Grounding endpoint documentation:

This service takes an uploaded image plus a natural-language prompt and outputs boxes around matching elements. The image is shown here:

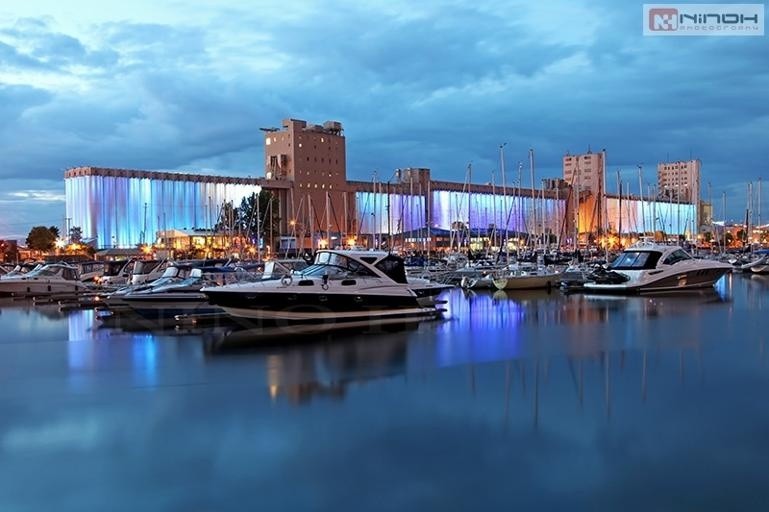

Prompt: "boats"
[0,141,769,338]
